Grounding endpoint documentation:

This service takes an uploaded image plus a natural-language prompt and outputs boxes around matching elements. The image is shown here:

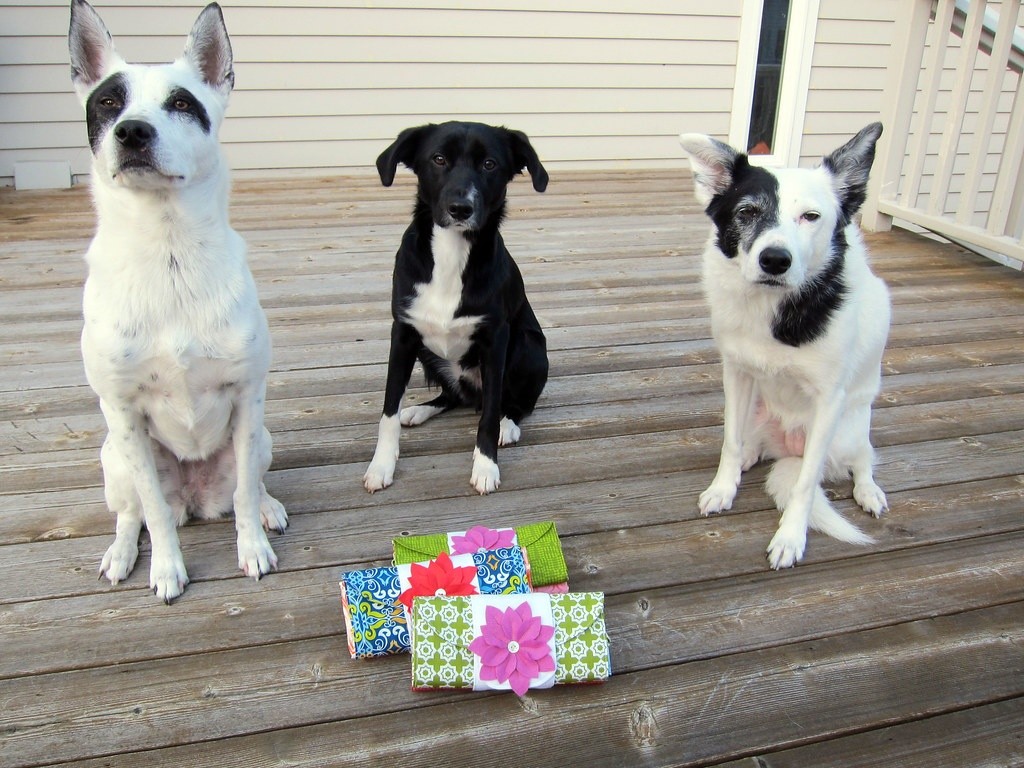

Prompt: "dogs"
[65,1,291,603]
[673,122,891,572]
[358,121,551,495]
